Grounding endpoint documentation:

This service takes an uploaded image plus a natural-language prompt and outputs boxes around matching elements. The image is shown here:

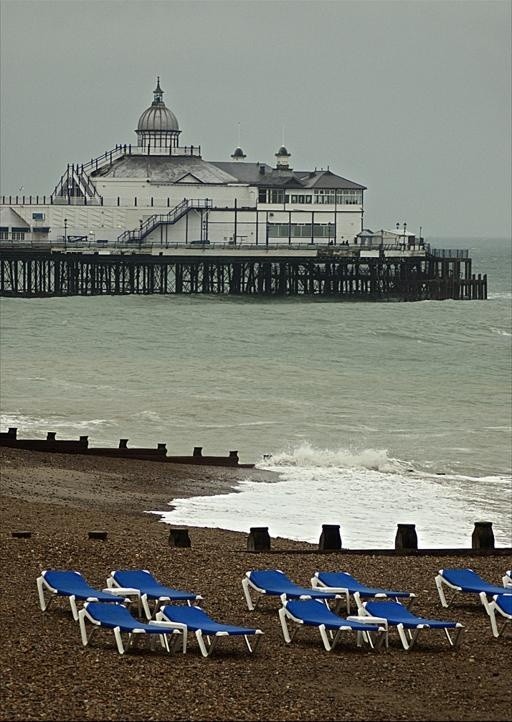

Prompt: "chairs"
[241,569,466,652]
[36,570,265,658]
[435,567,512,640]
